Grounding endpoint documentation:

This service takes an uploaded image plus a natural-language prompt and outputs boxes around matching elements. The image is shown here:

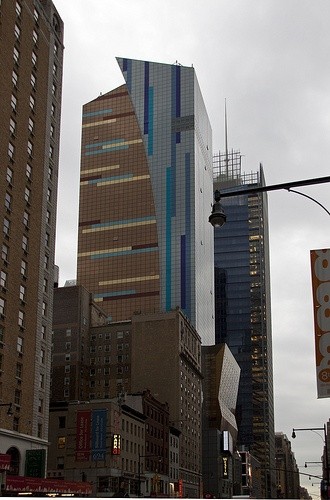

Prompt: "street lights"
[291,424,329,472]
[139,454,163,497]
